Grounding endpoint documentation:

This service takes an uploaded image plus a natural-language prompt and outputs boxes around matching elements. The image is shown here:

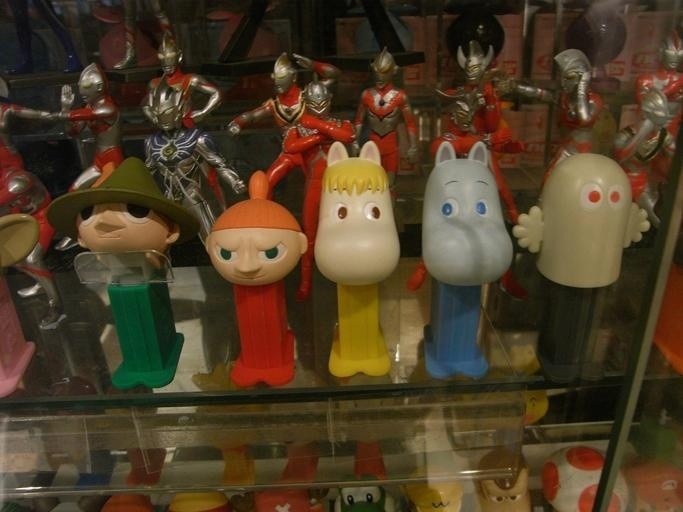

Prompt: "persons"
[1,1,683,301]
